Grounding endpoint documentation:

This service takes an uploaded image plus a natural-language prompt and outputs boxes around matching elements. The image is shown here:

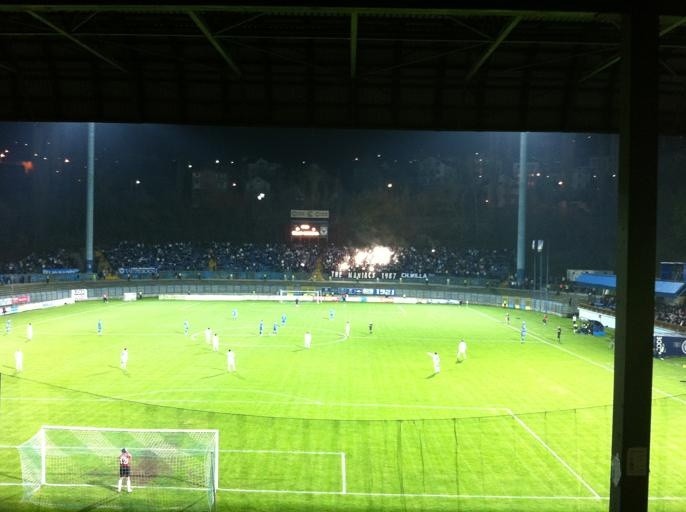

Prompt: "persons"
[117,448,133,493]
[0,240,686,376]
